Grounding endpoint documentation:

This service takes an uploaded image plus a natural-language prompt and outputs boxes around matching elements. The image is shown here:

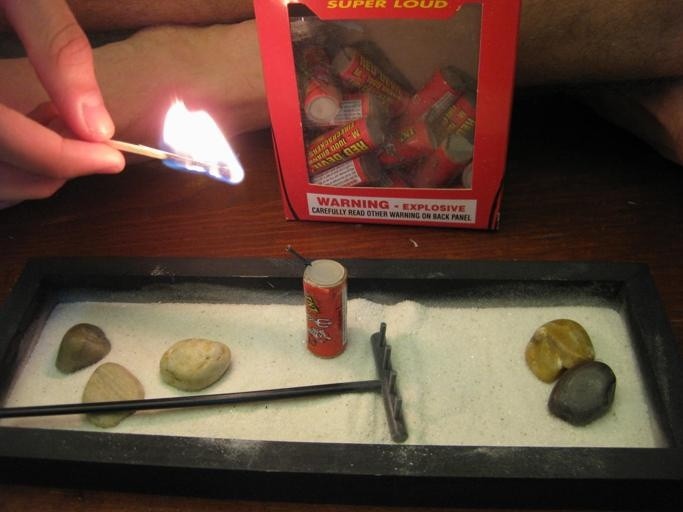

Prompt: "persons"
[0,1,126,213]
